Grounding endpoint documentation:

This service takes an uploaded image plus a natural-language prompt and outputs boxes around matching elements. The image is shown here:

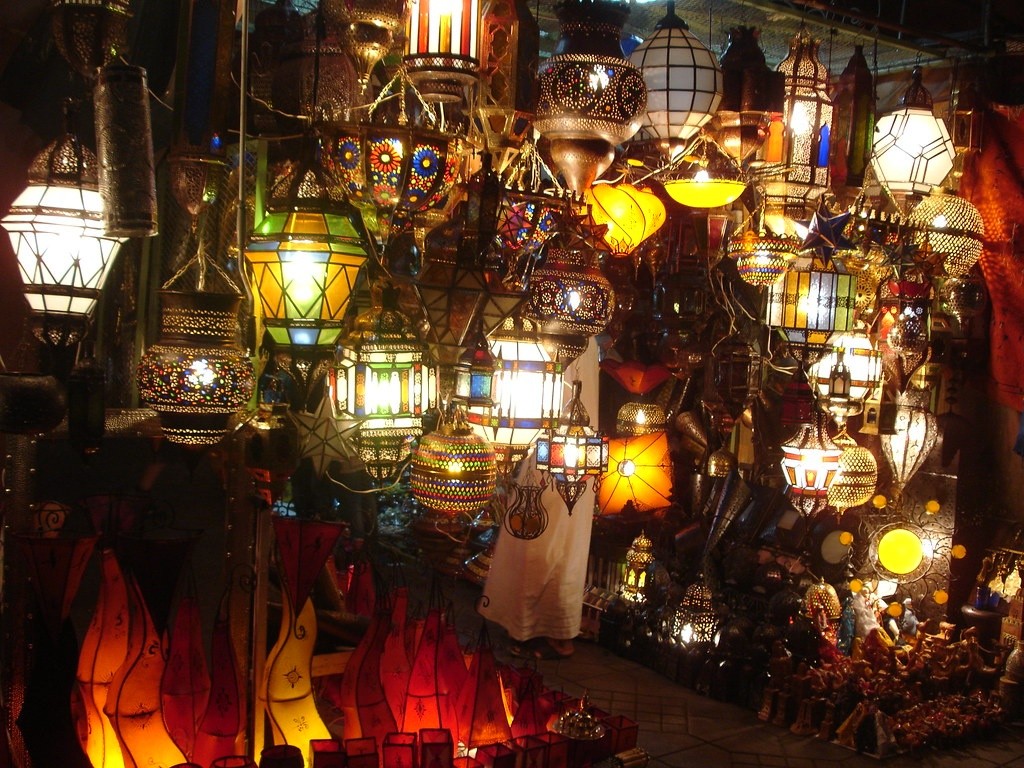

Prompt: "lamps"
[0,0,986,768]
